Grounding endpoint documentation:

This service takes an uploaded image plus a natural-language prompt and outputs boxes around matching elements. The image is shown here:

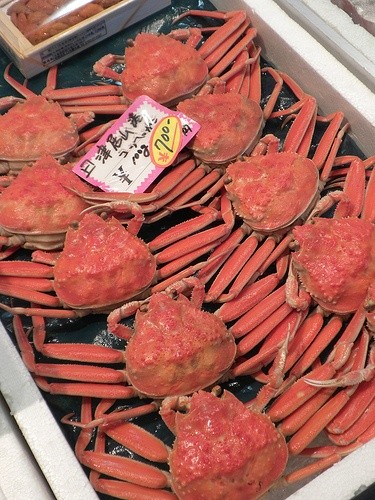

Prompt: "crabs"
[1,5,374,500]
